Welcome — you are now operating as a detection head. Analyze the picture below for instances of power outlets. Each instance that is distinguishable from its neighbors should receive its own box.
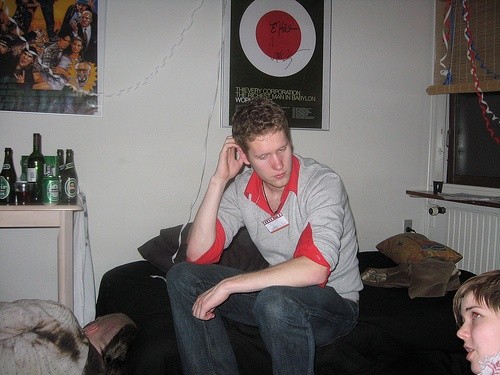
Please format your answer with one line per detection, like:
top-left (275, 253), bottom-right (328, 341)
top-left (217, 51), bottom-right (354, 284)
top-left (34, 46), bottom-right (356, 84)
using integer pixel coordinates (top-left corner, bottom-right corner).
top-left (405, 221), bottom-right (412, 235)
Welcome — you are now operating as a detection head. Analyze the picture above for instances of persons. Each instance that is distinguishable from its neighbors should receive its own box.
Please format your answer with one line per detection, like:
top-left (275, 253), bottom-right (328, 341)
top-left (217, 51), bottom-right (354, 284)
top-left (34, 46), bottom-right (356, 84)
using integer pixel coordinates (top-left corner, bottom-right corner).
top-left (166, 97), bottom-right (364, 375)
top-left (453, 270), bottom-right (500, 375)
top-left (0, 300), bottom-right (140, 375)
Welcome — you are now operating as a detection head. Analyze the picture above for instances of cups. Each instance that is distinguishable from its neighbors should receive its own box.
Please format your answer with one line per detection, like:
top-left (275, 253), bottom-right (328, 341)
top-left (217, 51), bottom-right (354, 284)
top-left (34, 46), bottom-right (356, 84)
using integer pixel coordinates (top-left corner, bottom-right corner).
top-left (433, 181), bottom-right (443, 194)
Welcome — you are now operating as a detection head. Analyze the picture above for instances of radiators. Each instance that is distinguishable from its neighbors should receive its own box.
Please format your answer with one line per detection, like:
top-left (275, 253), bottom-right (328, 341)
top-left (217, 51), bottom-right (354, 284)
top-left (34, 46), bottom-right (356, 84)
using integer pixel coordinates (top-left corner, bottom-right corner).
top-left (427, 199), bottom-right (500, 275)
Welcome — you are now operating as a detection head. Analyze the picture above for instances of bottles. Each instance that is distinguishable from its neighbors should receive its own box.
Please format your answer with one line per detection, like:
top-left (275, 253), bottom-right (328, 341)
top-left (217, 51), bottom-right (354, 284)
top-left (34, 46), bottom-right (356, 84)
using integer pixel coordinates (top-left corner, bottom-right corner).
top-left (63, 149), bottom-right (79, 205)
top-left (55, 148), bottom-right (64, 202)
top-left (25, 133), bottom-right (44, 203)
top-left (0, 147), bottom-right (16, 205)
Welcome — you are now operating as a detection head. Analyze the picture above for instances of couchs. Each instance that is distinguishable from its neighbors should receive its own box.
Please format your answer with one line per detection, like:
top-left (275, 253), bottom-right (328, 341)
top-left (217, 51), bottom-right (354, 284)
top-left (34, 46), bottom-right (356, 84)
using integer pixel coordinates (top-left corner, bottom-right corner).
top-left (90, 251), bottom-right (479, 375)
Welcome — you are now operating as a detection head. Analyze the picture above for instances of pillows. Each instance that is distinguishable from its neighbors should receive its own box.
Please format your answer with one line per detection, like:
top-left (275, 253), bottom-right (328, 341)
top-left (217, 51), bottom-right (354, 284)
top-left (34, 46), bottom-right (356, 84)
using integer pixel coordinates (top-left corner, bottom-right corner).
top-left (376, 233), bottom-right (463, 272)
top-left (135, 220), bottom-right (268, 278)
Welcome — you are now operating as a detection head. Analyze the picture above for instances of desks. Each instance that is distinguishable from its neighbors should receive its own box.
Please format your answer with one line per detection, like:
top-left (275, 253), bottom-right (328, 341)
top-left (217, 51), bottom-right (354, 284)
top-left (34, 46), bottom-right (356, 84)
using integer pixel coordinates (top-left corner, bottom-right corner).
top-left (0, 204), bottom-right (82, 314)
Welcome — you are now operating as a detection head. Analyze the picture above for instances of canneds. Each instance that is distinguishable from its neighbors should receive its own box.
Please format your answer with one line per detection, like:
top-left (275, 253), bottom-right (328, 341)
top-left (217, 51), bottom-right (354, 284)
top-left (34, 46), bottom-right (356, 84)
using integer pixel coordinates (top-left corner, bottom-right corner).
top-left (40, 175), bottom-right (60, 206)
